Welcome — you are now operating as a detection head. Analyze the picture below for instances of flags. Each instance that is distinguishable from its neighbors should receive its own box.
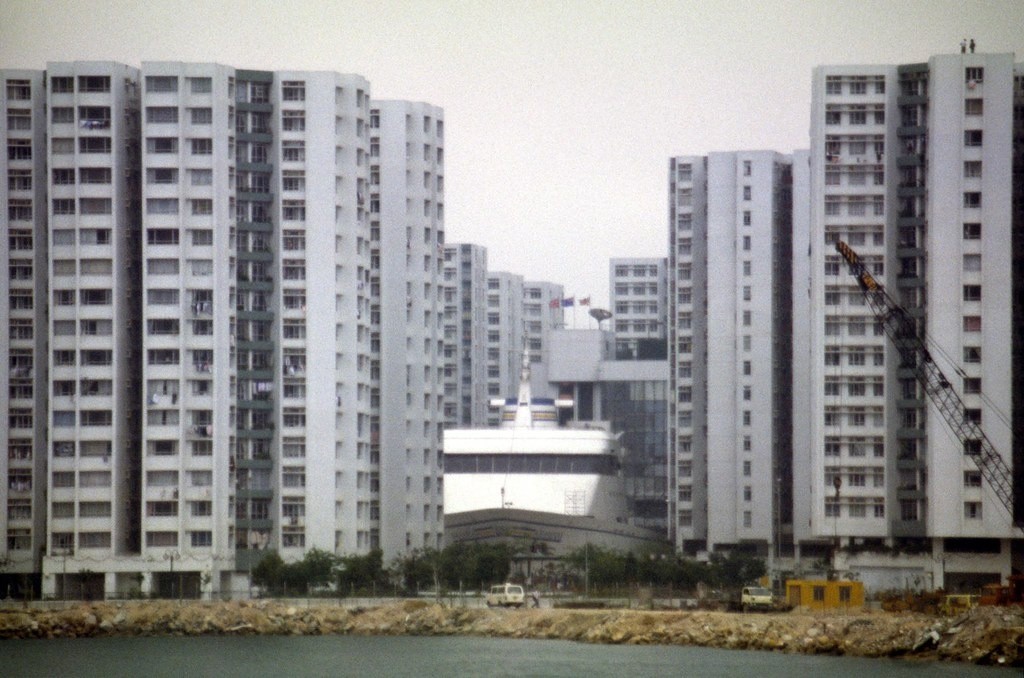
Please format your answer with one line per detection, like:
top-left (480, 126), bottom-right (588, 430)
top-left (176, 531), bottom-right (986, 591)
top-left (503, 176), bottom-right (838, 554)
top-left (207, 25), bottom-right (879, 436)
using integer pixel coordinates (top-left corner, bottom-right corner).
top-left (579, 297), bottom-right (590, 306)
top-left (561, 296), bottom-right (573, 306)
top-left (549, 299), bottom-right (559, 308)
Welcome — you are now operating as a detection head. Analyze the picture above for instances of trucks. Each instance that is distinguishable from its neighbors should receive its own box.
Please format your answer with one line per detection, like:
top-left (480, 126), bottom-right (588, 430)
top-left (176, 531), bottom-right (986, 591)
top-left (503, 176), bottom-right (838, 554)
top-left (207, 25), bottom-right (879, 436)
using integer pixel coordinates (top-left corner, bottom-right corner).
top-left (728, 585), bottom-right (773, 611)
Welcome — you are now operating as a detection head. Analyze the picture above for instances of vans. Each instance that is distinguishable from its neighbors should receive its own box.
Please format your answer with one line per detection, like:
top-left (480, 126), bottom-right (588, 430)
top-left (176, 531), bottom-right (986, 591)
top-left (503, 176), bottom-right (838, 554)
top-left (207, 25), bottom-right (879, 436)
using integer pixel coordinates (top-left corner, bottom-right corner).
top-left (485, 583), bottom-right (524, 608)
